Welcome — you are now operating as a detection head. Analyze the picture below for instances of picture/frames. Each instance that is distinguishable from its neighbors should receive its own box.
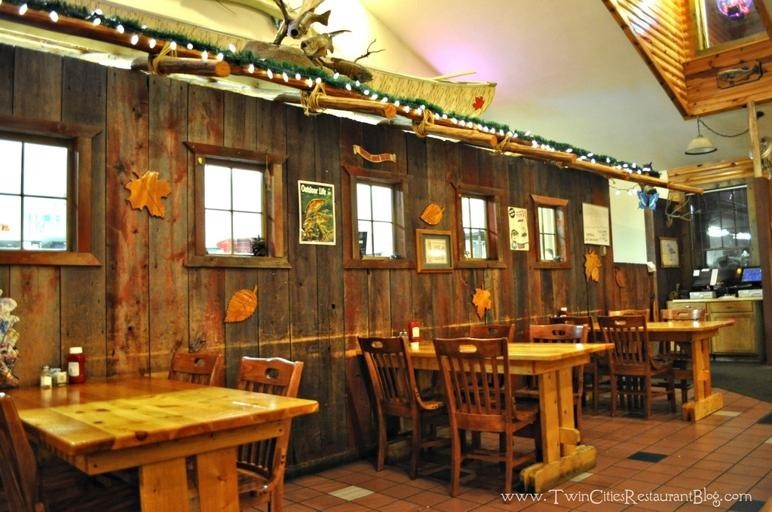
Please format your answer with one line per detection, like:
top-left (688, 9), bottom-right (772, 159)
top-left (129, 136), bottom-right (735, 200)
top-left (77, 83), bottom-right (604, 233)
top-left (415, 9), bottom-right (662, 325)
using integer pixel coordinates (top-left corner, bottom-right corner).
top-left (413, 226), bottom-right (456, 275)
top-left (657, 235), bottom-right (683, 270)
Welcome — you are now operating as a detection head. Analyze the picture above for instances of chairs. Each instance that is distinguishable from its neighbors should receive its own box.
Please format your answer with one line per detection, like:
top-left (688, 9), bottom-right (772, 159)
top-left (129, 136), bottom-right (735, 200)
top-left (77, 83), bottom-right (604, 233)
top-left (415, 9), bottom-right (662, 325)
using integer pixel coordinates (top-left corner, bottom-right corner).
top-left (462, 305), bottom-right (707, 445)
top-left (0, 391), bottom-right (138, 510)
top-left (355, 333), bottom-right (470, 480)
top-left (108, 350), bottom-right (225, 482)
top-left (231, 355), bottom-right (306, 512)
top-left (427, 337), bottom-right (544, 504)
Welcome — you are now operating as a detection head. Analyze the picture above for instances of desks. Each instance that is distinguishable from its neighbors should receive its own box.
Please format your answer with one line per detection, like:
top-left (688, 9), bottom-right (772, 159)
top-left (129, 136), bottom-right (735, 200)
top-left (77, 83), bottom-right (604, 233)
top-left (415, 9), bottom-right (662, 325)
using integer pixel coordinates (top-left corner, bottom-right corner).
top-left (577, 320), bottom-right (737, 423)
top-left (343, 340), bottom-right (617, 494)
top-left (3, 374), bottom-right (320, 512)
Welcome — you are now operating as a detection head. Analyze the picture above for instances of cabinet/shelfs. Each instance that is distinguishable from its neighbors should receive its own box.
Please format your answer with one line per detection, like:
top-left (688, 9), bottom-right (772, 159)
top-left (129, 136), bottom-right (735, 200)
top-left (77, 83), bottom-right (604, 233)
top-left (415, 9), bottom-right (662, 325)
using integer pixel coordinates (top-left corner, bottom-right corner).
top-left (665, 297), bottom-right (764, 356)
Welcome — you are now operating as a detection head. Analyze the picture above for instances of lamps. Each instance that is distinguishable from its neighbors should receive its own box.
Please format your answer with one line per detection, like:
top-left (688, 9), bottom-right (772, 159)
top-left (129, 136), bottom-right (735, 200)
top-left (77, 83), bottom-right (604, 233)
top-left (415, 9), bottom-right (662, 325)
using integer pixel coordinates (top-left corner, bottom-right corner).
top-left (681, 113), bottom-right (750, 157)
top-left (1, 1), bottom-right (659, 200)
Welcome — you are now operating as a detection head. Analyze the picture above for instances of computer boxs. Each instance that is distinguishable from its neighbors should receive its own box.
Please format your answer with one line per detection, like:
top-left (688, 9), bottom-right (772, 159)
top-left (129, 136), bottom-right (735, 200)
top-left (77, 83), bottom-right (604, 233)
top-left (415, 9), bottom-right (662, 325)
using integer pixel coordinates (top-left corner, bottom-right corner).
top-left (689, 292), bottom-right (717, 298)
top-left (738, 289), bottom-right (763, 298)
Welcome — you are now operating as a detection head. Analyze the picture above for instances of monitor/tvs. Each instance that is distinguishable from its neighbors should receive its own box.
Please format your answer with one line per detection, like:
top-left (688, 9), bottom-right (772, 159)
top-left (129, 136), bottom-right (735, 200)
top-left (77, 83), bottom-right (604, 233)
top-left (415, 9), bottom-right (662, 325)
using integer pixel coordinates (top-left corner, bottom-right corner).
top-left (689, 268), bottom-right (718, 290)
top-left (739, 266), bottom-right (762, 289)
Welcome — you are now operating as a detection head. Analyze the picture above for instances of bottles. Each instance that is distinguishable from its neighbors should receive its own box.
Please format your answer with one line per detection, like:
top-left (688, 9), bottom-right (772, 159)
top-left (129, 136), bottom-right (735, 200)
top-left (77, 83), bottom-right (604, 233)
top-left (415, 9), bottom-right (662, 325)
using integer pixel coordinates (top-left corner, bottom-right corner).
top-left (560, 307), bottom-right (569, 324)
top-left (399, 330), bottom-right (409, 351)
top-left (39, 366), bottom-right (52, 390)
top-left (40, 388), bottom-right (53, 408)
